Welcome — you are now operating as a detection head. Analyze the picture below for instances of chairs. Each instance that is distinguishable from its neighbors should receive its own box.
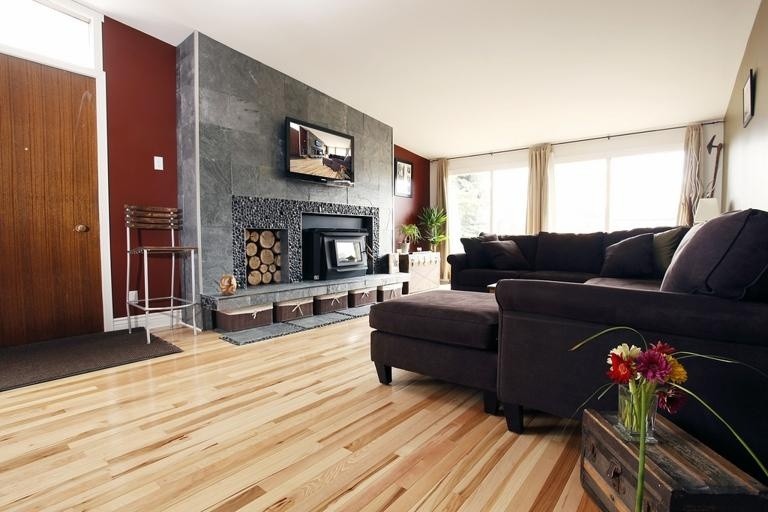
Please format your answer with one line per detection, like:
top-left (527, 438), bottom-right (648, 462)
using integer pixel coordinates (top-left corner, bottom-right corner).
top-left (123, 205), bottom-right (199, 344)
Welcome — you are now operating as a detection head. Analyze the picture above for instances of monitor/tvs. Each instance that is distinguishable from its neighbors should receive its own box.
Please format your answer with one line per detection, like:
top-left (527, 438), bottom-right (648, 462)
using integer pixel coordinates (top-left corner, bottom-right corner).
top-left (333, 239), bottom-right (364, 266)
top-left (284, 116), bottom-right (354, 191)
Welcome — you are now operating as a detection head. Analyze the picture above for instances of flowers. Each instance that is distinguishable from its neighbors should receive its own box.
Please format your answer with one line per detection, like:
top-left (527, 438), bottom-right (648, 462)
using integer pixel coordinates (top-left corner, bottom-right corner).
top-left (607, 341), bottom-right (686, 414)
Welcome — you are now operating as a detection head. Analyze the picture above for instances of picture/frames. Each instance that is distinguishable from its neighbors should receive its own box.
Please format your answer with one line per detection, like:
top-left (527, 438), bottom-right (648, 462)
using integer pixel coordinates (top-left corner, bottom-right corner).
top-left (394, 158), bottom-right (413, 198)
top-left (743, 68), bottom-right (753, 128)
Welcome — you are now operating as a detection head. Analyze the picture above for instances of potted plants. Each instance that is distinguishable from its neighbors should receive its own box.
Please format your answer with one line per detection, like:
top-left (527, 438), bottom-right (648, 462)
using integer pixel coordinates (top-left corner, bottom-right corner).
top-left (399, 224), bottom-right (425, 253)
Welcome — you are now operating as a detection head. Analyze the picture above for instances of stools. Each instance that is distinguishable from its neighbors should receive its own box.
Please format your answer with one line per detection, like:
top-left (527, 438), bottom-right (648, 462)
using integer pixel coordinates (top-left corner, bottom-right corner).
top-left (369, 290), bottom-right (498, 415)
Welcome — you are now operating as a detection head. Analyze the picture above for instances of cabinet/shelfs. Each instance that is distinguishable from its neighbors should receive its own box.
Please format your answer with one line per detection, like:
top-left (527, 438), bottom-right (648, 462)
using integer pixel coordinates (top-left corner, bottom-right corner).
top-left (396, 252), bottom-right (441, 294)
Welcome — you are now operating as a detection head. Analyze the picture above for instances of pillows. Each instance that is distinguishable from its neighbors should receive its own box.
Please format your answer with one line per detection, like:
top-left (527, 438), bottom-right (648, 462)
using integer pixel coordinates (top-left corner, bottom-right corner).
top-left (653, 226), bottom-right (682, 271)
top-left (460, 234), bottom-right (498, 265)
top-left (482, 240), bottom-right (531, 270)
top-left (601, 233), bottom-right (653, 277)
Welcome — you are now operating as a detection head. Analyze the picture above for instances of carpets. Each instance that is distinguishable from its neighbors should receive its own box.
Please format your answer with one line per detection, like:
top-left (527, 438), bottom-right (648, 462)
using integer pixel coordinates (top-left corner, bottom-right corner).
top-left (0, 327), bottom-right (183, 393)
top-left (219, 303), bottom-right (376, 346)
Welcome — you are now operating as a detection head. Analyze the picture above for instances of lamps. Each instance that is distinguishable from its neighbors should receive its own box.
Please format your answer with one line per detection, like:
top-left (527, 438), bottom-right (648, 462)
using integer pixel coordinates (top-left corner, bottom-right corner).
top-left (695, 198), bottom-right (720, 223)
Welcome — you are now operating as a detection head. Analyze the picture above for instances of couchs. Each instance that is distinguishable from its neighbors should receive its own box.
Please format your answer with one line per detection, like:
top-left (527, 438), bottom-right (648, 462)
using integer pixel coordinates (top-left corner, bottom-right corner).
top-left (447, 209), bottom-right (768, 432)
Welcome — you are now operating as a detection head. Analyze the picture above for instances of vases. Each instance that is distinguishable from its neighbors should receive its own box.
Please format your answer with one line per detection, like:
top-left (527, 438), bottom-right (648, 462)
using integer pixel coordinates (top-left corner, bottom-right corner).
top-left (614, 382), bottom-right (659, 442)
